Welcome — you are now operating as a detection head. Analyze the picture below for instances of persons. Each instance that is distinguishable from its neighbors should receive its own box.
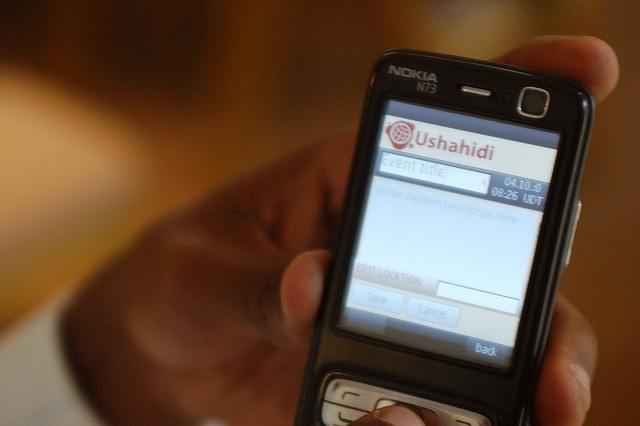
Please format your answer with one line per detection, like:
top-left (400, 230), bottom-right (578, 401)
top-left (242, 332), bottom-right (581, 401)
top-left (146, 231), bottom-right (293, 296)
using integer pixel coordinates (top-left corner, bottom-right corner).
top-left (1, 34), bottom-right (620, 426)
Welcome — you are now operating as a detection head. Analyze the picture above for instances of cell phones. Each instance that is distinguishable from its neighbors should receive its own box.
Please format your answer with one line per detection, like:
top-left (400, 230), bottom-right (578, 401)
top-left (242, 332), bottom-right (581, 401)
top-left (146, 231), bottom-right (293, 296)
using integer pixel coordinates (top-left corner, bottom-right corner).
top-left (294, 48), bottom-right (596, 426)
